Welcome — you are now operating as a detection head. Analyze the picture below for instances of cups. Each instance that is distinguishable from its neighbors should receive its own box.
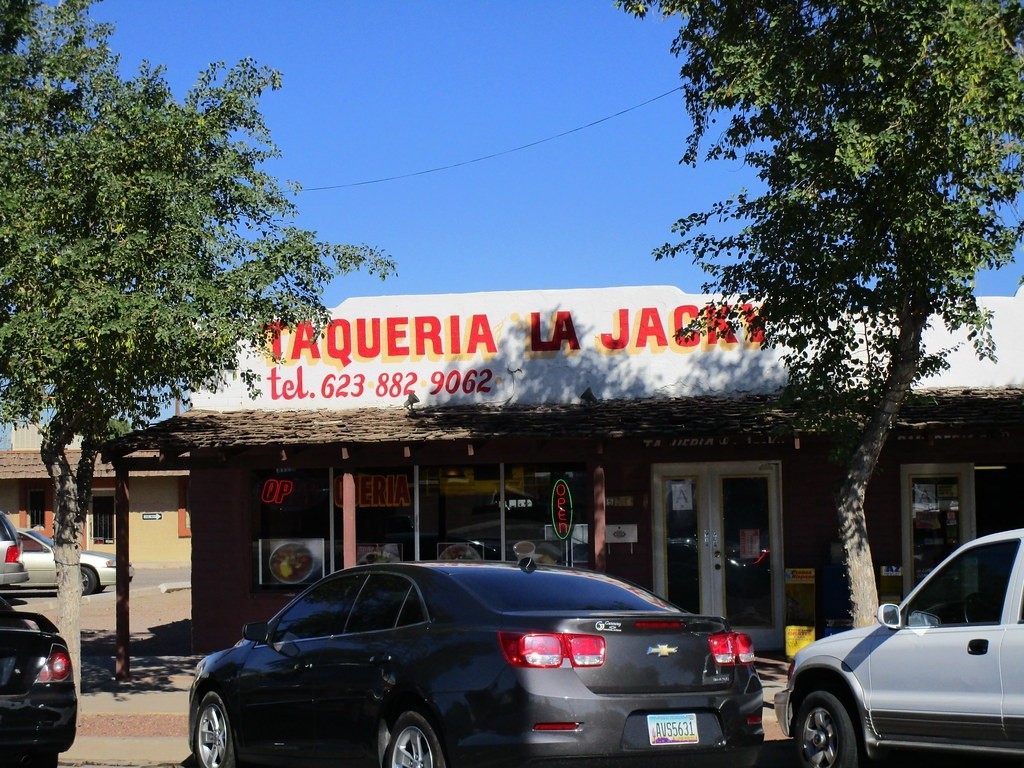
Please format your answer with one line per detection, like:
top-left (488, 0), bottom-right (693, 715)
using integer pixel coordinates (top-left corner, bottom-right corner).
top-left (513, 541), bottom-right (536, 561)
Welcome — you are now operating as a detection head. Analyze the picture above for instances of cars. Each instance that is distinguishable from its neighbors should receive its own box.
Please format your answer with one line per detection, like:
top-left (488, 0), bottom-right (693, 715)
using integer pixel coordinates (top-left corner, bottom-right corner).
top-left (0, 512), bottom-right (29, 585)
top-left (0, 598), bottom-right (78, 768)
top-left (188, 563), bottom-right (765, 768)
top-left (16, 530), bottom-right (134, 595)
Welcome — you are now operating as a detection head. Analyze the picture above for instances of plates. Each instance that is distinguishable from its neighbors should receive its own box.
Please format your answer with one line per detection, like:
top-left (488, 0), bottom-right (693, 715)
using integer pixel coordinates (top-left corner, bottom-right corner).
top-left (357, 551), bottom-right (400, 566)
top-left (268, 542), bottom-right (315, 584)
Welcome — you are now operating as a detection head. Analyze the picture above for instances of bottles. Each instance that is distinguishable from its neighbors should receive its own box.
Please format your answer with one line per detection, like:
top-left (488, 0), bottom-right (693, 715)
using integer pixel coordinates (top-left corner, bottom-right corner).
top-left (439, 544), bottom-right (481, 560)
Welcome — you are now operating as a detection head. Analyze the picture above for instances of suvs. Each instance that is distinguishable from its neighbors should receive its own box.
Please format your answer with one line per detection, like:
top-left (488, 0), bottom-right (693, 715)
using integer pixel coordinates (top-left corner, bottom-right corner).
top-left (776, 529), bottom-right (1022, 768)
